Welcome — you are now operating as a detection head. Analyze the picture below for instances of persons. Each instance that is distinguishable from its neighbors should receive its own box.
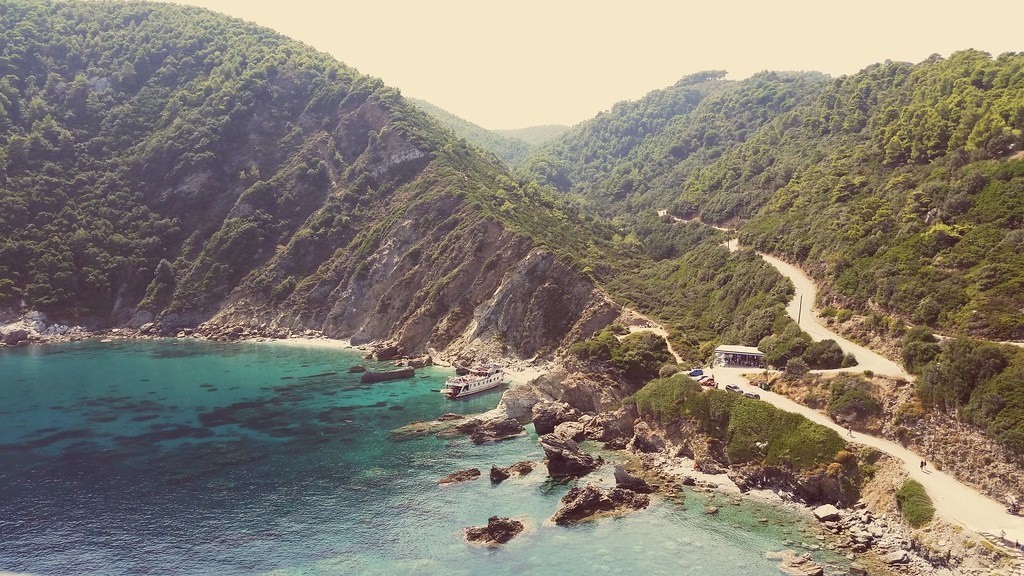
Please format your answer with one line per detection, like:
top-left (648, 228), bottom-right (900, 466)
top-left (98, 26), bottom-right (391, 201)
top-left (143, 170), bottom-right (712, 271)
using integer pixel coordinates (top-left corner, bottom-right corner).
top-left (920, 460), bottom-right (924, 471)
top-left (846, 426), bottom-right (852, 437)
top-left (923, 460), bottom-right (927, 471)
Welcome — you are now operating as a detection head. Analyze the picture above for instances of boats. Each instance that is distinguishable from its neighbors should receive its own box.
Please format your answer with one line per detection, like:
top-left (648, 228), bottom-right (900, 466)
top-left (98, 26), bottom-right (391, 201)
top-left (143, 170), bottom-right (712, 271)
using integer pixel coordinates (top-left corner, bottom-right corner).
top-left (442, 363), bottom-right (503, 399)
